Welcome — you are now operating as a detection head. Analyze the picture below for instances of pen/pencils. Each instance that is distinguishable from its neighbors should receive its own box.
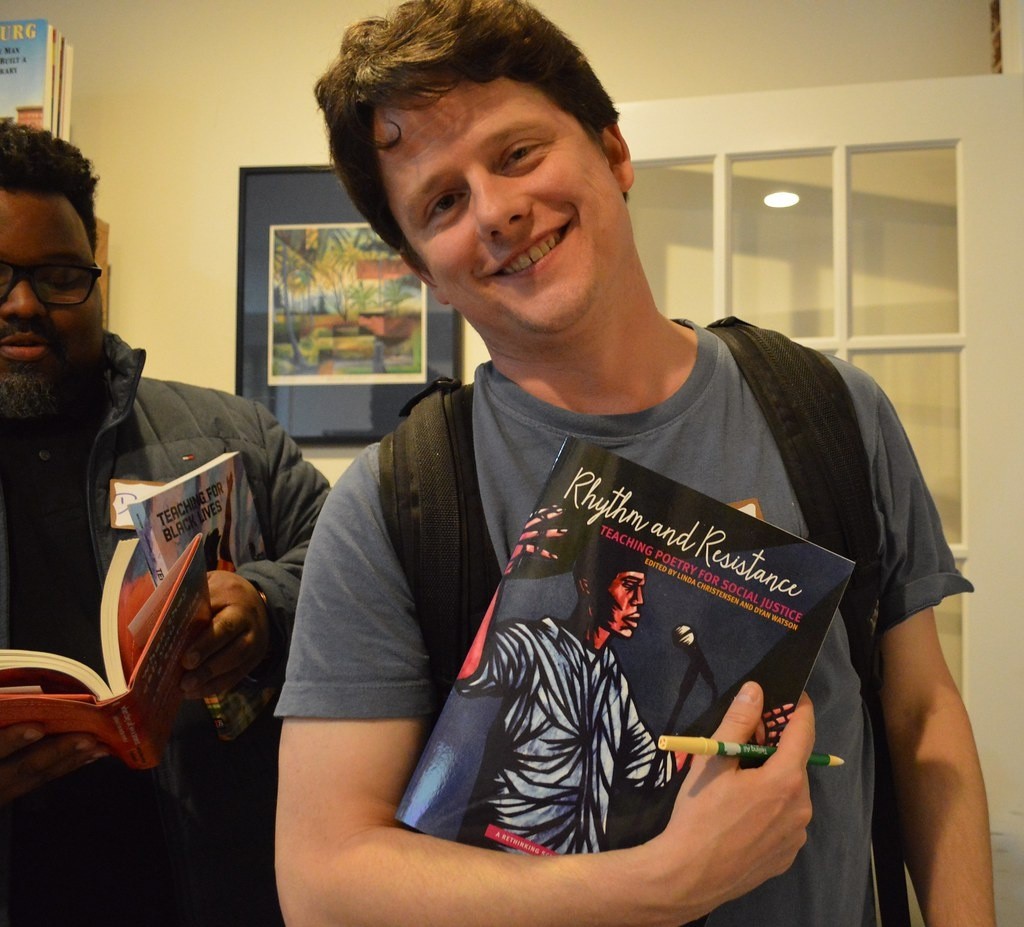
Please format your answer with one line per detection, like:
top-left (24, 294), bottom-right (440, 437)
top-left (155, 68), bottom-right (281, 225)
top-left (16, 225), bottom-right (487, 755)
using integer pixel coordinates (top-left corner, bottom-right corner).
top-left (658, 735), bottom-right (845, 768)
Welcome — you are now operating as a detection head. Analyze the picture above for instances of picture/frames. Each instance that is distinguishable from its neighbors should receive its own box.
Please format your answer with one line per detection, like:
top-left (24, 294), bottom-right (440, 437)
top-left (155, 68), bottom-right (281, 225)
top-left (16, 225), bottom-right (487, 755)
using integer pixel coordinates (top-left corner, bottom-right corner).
top-left (234, 164), bottom-right (463, 447)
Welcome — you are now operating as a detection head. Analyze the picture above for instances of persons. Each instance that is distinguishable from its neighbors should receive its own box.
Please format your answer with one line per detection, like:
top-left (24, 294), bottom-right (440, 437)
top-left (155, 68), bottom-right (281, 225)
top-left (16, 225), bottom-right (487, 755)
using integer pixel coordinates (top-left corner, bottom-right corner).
top-left (0, 119), bottom-right (334, 927)
top-left (273, 1), bottom-right (997, 927)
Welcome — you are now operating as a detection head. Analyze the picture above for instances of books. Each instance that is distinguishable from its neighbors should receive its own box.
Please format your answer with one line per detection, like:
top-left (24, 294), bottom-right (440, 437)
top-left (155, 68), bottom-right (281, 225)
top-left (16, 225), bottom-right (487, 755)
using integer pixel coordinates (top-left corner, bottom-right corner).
top-left (0, 441), bottom-right (280, 770)
top-left (397, 437), bottom-right (854, 927)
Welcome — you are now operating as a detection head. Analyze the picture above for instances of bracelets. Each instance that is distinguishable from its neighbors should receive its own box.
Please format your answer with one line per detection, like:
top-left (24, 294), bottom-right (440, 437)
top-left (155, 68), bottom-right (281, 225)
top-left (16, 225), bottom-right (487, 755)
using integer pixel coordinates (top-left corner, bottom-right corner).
top-left (258, 591), bottom-right (269, 607)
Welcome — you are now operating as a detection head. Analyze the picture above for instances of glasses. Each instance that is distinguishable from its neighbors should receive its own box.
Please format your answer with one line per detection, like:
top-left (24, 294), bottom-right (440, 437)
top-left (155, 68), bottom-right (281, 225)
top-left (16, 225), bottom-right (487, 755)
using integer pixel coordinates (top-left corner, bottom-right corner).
top-left (0, 260), bottom-right (102, 305)
